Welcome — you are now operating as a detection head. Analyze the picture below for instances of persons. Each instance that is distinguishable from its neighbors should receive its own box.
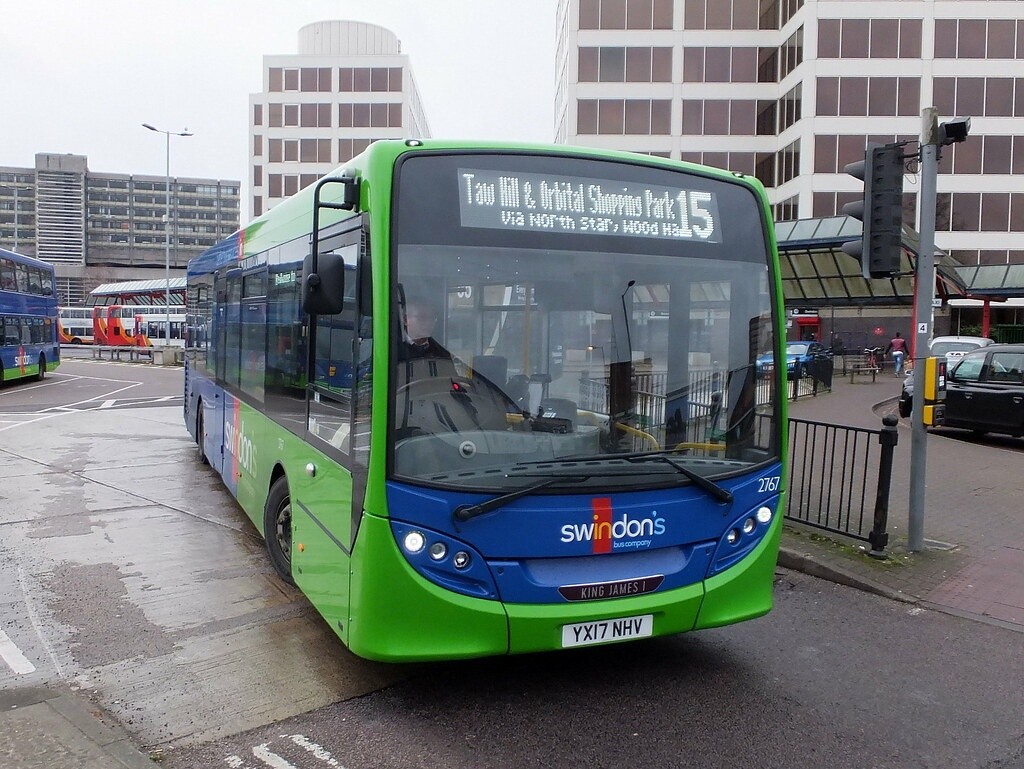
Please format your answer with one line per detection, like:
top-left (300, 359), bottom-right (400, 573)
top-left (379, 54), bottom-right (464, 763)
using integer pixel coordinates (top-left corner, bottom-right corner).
top-left (379, 292), bottom-right (483, 448)
top-left (883, 331), bottom-right (912, 379)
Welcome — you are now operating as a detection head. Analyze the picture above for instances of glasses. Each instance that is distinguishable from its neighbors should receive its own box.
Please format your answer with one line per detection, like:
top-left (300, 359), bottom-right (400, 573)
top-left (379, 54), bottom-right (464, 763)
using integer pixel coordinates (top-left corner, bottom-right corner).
top-left (407, 314), bottom-right (438, 323)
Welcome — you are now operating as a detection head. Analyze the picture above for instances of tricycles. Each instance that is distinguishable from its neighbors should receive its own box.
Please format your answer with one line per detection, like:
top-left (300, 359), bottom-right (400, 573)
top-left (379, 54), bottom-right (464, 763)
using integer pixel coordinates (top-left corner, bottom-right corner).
top-left (847, 347), bottom-right (883, 383)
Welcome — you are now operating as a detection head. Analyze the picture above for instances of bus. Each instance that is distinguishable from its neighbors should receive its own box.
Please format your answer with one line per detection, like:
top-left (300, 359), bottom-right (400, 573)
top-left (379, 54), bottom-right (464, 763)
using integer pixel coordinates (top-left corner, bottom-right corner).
top-left (0, 247), bottom-right (61, 384)
top-left (133, 312), bottom-right (186, 352)
top-left (55, 306), bottom-right (94, 345)
top-left (92, 305), bottom-right (109, 346)
top-left (106, 303), bottom-right (187, 345)
top-left (183, 133), bottom-right (796, 668)
top-left (214, 257), bottom-right (373, 402)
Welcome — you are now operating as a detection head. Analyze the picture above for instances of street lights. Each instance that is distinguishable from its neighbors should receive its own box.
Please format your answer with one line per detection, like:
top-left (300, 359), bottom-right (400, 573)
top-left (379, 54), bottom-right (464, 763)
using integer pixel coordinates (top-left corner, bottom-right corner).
top-left (587, 343), bottom-right (607, 374)
top-left (620, 280), bottom-right (637, 366)
top-left (142, 122), bottom-right (196, 347)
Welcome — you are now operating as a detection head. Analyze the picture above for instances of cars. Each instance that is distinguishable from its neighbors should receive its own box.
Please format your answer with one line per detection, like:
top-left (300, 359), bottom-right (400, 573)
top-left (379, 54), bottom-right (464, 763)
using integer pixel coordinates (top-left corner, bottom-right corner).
top-left (755, 339), bottom-right (830, 382)
top-left (903, 336), bottom-right (994, 394)
top-left (898, 344), bottom-right (1024, 447)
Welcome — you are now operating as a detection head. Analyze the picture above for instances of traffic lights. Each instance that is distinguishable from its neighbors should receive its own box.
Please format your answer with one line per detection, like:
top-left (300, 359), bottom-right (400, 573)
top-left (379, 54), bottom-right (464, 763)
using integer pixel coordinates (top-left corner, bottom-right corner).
top-left (844, 141), bottom-right (904, 282)
